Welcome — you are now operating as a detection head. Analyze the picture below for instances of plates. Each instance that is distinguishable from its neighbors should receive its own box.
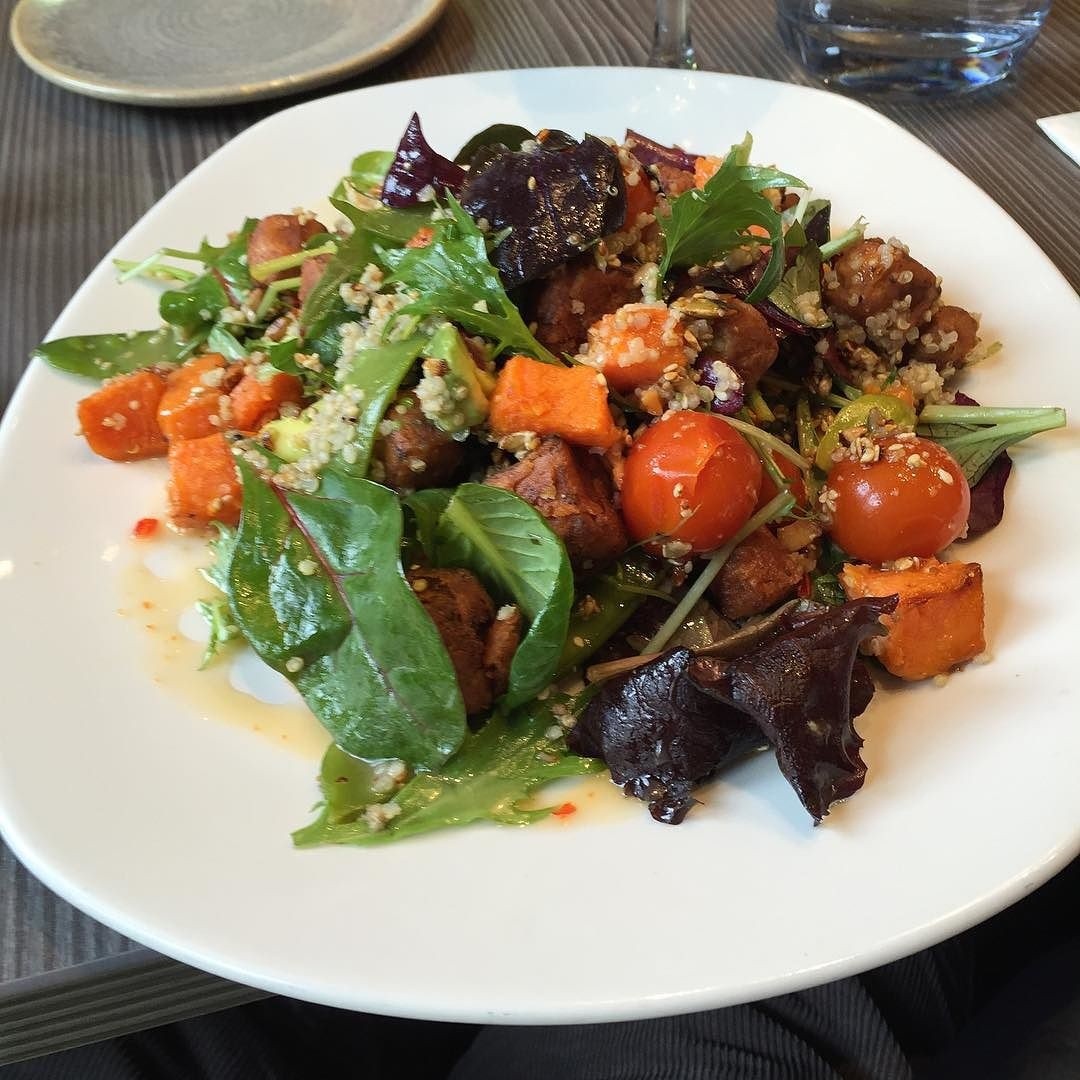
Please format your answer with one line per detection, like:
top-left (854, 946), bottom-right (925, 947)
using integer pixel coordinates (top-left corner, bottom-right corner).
top-left (7, 0), bottom-right (449, 108)
top-left (0, 65), bottom-right (1080, 1026)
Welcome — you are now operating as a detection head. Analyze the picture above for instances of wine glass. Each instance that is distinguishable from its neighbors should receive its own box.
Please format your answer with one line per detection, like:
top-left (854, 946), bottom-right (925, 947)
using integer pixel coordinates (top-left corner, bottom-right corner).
top-left (644, 0), bottom-right (703, 71)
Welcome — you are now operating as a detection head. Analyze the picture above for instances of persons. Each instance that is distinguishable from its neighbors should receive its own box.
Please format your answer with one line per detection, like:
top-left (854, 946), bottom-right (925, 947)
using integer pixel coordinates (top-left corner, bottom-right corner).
top-left (0, 939), bottom-right (972, 1080)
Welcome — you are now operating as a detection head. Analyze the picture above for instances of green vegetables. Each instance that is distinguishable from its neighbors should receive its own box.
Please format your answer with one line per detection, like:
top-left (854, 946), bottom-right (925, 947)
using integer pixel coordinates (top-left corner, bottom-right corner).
top-left (21, 123), bottom-right (1068, 870)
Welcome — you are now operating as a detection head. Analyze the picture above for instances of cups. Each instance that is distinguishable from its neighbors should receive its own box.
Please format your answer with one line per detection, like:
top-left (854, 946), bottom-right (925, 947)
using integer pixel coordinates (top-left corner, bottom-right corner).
top-left (774, 0), bottom-right (1050, 97)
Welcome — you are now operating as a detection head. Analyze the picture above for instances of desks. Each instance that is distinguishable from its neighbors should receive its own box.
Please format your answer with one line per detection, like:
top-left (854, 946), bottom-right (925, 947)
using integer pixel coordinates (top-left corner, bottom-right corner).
top-left (1, 0), bottom-right (1080, 1070)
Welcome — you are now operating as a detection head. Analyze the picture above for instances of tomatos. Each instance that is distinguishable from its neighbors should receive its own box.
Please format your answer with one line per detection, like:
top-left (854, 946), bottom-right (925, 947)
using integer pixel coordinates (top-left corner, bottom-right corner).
top-left (816, 433), bottom-right (971, 566)
top-left (759, 437), bottom-right (807, 525)
top-left (615, 409), bottom-right (759, 557)
